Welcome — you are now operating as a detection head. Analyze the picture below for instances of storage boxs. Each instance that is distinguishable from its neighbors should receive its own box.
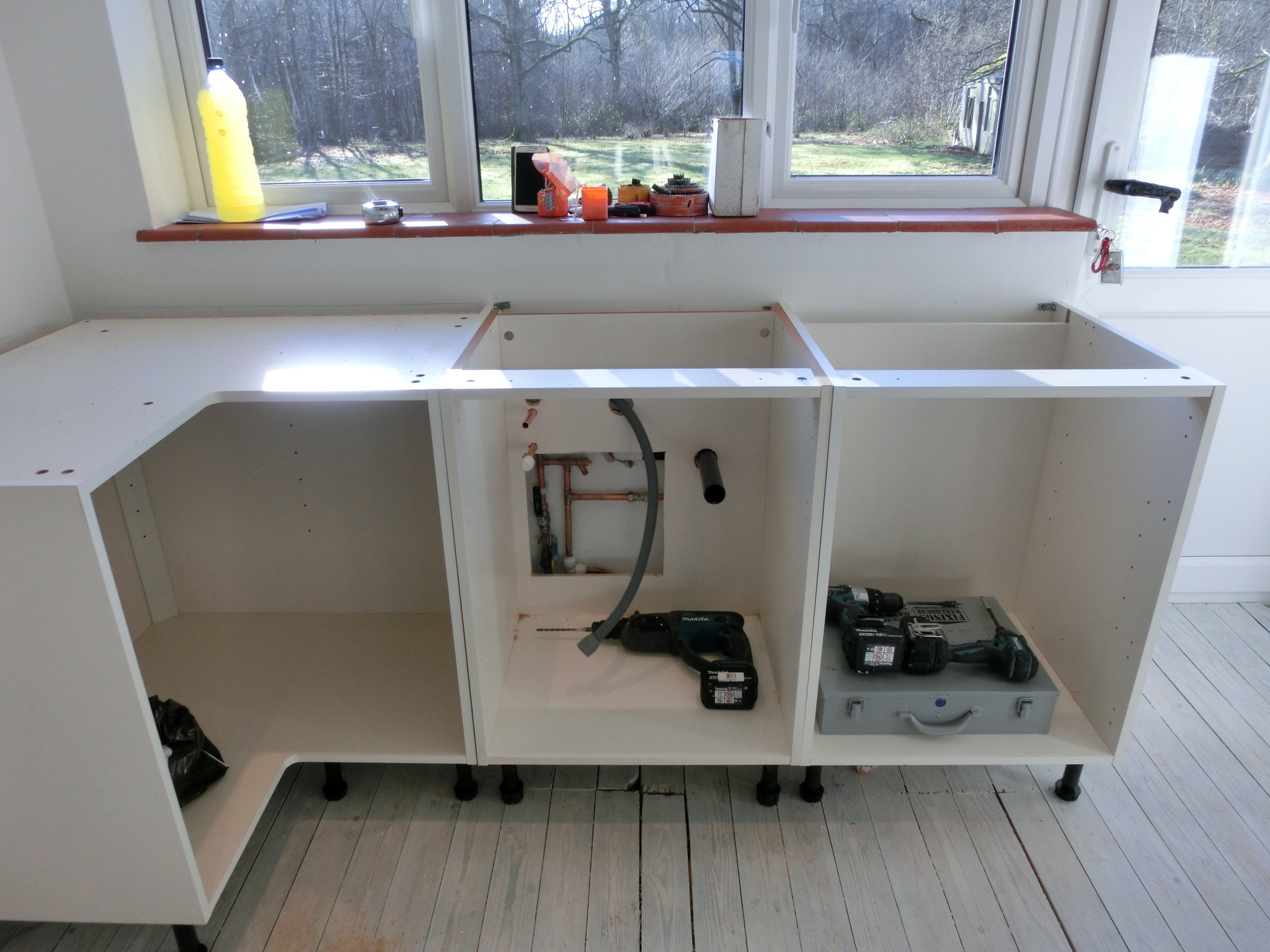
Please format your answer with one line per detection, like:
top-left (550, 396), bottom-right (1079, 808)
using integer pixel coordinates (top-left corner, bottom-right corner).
top-left (709, 115), bottom-right (765, 216)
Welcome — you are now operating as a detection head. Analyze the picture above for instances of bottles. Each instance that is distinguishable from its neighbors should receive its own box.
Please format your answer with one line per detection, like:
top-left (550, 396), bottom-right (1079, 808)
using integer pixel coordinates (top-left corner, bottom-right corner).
top-left (196, 57), bottom-right (266, 222)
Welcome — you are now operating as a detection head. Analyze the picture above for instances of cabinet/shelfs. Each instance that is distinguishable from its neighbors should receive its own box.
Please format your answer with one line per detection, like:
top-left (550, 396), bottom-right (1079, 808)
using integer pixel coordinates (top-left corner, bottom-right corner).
top-left (434, 305), bottom-right (834, 808)
top-left (0, 304), bottom-right (491, 952)
top-left (794, 301), bottom-right (1226, 804)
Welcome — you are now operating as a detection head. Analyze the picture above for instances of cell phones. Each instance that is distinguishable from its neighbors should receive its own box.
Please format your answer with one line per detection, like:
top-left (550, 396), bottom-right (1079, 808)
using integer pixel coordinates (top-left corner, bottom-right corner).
top-left (512, 146), bottom-right (549, 213)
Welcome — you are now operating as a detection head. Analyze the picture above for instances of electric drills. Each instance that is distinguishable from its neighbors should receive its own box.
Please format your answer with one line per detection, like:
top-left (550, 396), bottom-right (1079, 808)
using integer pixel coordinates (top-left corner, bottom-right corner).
top-left (535, 609), bottom-right (759, 710)
top-left (842, 597), bottom-right (1039, 682)
top-left (825, 584), bottom-right (962, 623)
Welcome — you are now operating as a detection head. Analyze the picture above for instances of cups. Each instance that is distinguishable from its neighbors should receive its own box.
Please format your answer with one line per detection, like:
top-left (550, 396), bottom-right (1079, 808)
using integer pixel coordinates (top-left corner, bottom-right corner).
top-left (581, 186), bottom-right (609, 221)
